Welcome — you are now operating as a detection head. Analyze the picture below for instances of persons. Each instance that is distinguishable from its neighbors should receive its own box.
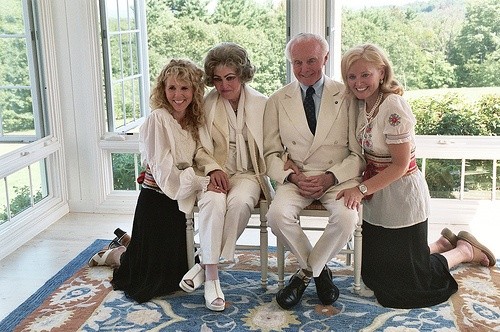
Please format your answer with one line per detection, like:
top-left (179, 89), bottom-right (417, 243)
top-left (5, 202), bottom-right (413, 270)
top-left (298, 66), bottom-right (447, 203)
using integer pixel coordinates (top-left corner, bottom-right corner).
top-left (179, 41), bottom-right (299, 311)
top-left (263, 32), bottom-right (367, 310)
top-left (88, 59), bottom-right (227, 303)
top-left (336, 44), bottom-right (496, 309)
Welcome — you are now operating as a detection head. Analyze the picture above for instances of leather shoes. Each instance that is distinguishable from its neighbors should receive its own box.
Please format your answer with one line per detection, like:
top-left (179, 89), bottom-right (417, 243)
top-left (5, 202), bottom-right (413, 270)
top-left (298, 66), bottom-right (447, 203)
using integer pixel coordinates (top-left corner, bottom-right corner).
top-left (276, 269), bottom-right (311, 310)
top-left (314, 264), bottom-right (339, 305)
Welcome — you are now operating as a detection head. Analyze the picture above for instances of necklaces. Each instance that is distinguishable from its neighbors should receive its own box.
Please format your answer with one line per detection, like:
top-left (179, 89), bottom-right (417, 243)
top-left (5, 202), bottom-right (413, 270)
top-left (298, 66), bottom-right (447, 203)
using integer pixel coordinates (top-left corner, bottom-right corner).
top-left (233, 107), bottom-right (238, 111)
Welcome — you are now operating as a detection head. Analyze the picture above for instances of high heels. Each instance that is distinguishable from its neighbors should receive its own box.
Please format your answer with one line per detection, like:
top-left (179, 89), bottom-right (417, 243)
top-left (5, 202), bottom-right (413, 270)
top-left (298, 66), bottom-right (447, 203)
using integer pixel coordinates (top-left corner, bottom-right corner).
top-left (109, 228), bottom-right (130, 249)
top-left (88, 241), bottom-right (122, 267)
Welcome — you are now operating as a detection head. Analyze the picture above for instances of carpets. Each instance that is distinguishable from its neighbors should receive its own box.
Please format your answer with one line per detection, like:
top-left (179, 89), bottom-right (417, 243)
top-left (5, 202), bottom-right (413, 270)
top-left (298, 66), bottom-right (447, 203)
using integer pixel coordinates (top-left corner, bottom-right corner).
top-left (0, 239), bottom-right (500, 332)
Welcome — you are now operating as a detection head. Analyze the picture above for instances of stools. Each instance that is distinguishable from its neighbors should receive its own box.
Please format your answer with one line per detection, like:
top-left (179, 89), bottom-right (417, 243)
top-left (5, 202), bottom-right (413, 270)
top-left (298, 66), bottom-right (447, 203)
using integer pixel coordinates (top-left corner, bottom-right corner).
top-left (185, 194), bottom-right (269, 287)
top-left (277, 201), bottom-right (364, 294)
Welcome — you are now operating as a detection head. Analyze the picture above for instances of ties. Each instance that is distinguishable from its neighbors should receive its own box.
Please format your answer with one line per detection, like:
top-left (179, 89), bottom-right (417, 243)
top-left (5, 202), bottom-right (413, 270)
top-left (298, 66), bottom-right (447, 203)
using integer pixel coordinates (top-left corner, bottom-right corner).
top-left (303, 87), bottom-right (317, 136)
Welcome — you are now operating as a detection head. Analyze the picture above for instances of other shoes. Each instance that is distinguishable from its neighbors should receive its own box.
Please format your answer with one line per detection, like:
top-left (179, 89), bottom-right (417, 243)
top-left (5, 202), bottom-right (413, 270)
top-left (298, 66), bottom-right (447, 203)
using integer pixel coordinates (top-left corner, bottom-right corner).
top-left (204, 278), bottom-right (225, 311)
top-left (179, 263), bottom-right (204, 292)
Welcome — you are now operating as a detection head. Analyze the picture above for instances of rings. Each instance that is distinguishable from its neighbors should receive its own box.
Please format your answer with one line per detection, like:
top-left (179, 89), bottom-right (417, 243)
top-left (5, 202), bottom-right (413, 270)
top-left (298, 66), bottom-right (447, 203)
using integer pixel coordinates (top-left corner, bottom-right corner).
top-left (215, 186), bottom-right (219, 189)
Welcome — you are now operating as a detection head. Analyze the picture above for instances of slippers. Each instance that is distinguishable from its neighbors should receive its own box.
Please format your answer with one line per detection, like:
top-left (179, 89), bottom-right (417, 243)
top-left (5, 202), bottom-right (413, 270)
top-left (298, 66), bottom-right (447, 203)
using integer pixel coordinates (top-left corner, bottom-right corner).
top-left (441, 228), bottom-right (458, 248)
top-left (458, 231), bottom-right (496, 266)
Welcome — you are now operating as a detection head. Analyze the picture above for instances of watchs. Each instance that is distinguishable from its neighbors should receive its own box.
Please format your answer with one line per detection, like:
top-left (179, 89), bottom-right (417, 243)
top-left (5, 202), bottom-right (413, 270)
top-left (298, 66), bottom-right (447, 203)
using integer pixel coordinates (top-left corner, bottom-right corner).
top-left (357, 183), bottom-right (368, 196)
